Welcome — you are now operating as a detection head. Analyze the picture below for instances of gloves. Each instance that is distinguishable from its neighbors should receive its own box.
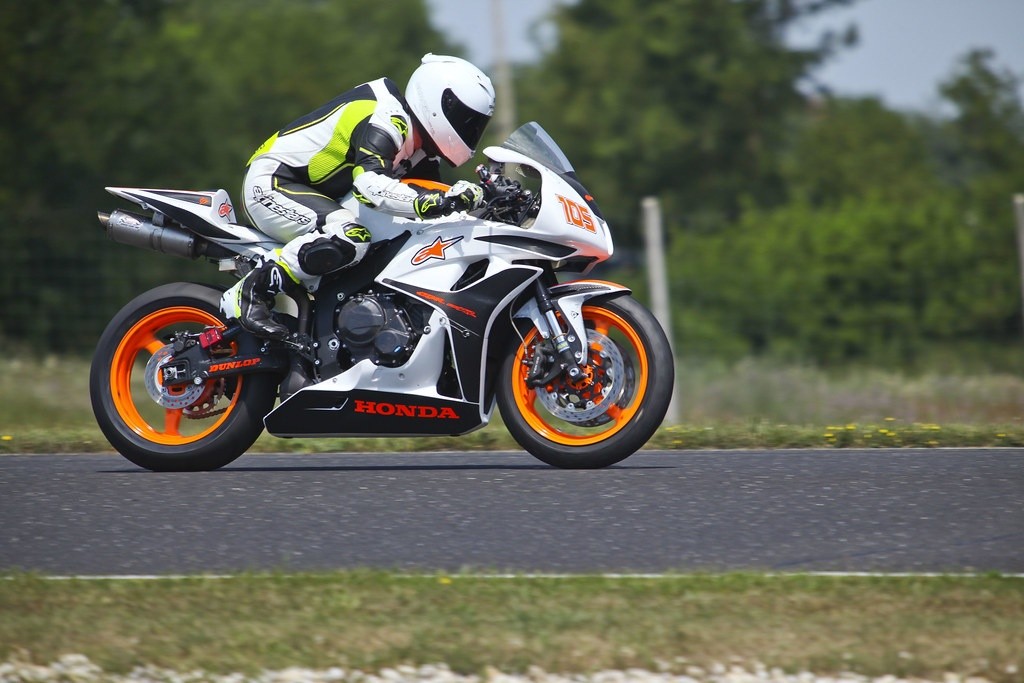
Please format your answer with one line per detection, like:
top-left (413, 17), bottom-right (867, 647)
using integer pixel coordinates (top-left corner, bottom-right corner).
top-left (413, 180), bottom-right (486, 221)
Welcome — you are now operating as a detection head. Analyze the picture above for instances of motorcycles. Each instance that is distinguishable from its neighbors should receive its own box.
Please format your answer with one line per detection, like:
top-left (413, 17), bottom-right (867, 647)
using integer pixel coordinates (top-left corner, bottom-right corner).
top-left (88, 120), bottom-right (675, 472)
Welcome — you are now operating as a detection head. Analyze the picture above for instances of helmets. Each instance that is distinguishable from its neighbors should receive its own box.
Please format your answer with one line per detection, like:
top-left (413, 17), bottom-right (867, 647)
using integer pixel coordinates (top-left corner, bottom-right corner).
top-left (403, 52), bottom-right (496, 168)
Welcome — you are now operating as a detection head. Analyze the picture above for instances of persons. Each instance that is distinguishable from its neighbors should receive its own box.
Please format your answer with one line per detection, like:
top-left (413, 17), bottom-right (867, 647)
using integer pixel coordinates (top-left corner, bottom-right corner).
top-left (219, 52), bottom-right (496, 340)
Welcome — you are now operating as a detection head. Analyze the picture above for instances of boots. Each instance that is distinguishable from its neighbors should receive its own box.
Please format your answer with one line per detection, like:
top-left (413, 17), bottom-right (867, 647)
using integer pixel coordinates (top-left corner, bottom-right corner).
top-left (219, 256), bottom-right (301, 342)
